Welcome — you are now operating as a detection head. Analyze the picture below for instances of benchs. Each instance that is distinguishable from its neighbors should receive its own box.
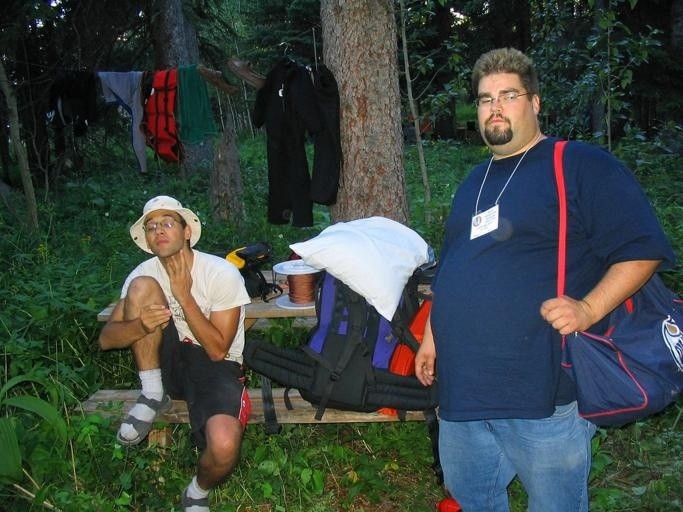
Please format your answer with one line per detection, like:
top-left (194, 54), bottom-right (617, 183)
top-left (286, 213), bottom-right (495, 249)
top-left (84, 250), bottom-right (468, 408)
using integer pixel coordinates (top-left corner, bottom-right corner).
top-left (77, 388), bottom-right (444, 496)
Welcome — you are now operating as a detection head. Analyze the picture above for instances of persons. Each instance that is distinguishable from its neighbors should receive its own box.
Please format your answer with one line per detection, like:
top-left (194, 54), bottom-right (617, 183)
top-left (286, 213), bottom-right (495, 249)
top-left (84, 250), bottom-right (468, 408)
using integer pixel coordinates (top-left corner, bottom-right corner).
top-left (98, 194), bottom-right (250, 512)
top-left (412, 47), bottom-right (671, 512)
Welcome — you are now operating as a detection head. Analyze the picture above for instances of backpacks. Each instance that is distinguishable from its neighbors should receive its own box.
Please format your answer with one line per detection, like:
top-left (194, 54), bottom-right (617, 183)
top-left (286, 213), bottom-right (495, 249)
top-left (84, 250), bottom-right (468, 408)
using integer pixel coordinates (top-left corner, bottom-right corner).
top-left (297, 266), bottom-right (422, 413)
top-left (222, 243), bottom-right (272, 303)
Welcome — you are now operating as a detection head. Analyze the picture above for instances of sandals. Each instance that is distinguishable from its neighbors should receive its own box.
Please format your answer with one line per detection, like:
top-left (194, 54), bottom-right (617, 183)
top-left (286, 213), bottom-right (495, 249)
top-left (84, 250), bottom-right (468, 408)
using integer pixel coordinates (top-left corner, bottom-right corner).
top-left (116, 387), bottom-right (173, 446)
top-left (181, 488), bottom-right (212, 512)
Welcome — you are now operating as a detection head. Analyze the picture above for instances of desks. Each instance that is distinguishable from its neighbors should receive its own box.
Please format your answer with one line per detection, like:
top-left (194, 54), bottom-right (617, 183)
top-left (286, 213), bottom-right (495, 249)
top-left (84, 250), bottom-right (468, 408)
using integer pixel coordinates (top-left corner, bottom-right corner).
top-left (96, 270), bottom-right (437, 334)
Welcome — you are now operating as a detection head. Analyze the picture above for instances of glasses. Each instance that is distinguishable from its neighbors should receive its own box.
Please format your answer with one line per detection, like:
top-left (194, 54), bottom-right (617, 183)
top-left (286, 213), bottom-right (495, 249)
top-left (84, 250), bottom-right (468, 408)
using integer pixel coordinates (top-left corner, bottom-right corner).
top-left (475, 91), bottom-right (535, 105)
top-left (142, 218), bottom-right (185, 229)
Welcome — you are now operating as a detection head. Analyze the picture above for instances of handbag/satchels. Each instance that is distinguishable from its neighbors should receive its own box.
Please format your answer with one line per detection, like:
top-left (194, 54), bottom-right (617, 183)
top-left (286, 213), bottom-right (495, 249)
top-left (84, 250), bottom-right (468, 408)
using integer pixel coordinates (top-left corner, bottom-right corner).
top-left (564, 276), bottom-right (682, 429)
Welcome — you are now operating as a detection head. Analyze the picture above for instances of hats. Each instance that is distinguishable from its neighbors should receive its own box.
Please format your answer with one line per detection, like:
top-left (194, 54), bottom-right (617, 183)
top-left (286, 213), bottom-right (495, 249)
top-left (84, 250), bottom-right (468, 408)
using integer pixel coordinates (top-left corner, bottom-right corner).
top-left (130, 195), bottom-right (202, 255)
